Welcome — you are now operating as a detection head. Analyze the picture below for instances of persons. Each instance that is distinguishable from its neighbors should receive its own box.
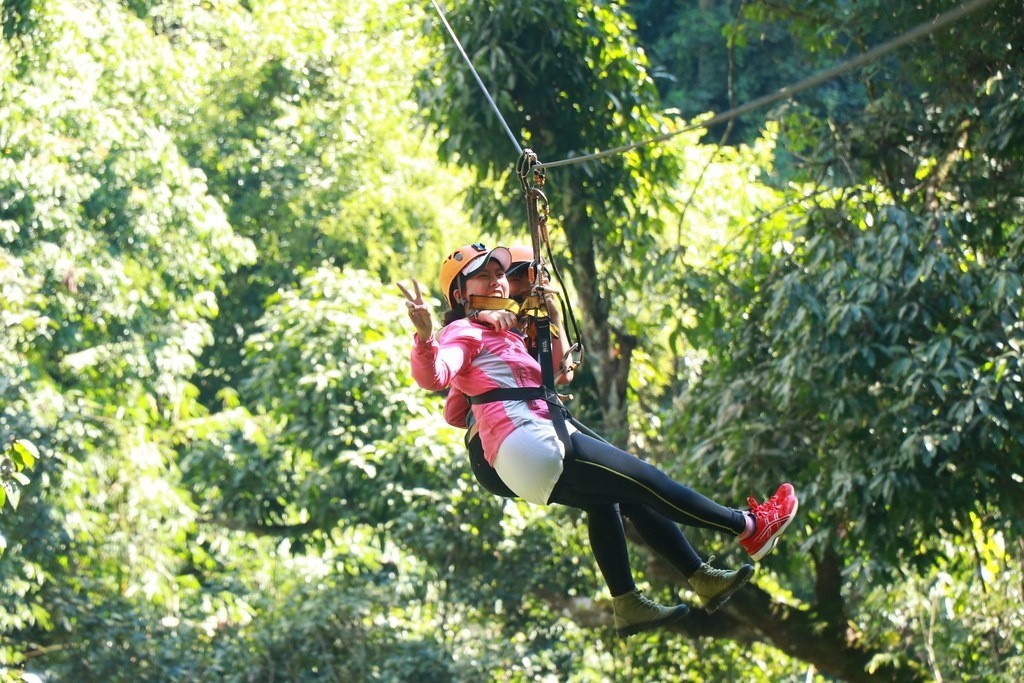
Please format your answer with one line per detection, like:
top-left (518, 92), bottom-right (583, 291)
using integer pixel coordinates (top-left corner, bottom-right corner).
top-left (444, 247), bottom-right (755, 639)
top-left (396, 243), bottom-right (799, 562)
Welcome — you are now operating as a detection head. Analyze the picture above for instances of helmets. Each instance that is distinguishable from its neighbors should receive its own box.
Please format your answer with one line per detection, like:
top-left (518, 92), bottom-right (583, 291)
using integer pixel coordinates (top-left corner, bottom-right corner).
top-left (439, 243), bottom-right (488, 310)
top-left (508, 246), bottom-right (543, 264)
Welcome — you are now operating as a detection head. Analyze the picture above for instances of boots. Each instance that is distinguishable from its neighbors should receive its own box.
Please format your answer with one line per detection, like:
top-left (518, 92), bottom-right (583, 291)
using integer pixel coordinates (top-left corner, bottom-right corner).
top-left (688, 555), bottom-right (755, 615)
top-left (612, 587), bottom-right (689, 639)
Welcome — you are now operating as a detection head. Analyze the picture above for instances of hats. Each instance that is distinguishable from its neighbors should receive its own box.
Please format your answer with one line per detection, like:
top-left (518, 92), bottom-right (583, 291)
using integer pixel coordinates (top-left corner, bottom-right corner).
top-left (451, 245), bottom-right (512, 303)
top-left (505, 261), bottom-right (551, 282)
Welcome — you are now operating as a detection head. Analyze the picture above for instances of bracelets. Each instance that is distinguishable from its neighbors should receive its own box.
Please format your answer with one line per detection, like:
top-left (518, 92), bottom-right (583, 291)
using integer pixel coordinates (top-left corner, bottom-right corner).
top-left (475, 310), bottom-right (483, 320)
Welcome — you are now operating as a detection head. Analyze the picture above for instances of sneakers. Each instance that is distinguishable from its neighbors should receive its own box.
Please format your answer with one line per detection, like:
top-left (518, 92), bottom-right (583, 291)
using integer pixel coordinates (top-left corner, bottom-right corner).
top-left (740, 495), bottom-right (799, 561)
top-left (747, 483), bottom-right (795, 556)
